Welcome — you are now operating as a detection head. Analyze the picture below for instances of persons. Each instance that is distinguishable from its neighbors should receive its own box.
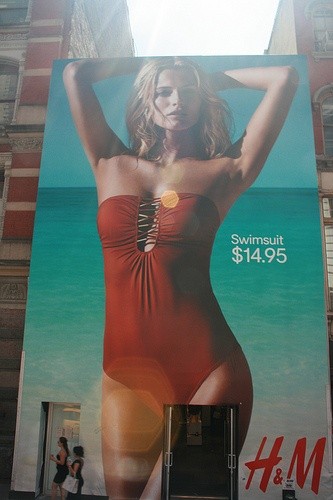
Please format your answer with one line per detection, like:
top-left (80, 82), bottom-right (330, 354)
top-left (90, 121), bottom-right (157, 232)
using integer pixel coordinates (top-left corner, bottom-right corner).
top-left (48, 436), bottom-right (71, 500)
top-left (67, 446), bottom-right (85, 500)
top-left (60, 57), bottom-right (299, 500)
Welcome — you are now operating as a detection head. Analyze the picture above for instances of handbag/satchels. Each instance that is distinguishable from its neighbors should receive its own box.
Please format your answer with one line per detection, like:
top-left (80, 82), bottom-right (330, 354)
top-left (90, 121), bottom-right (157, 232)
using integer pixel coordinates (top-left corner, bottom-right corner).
top-left (62, 469), bottom-right (79, 494)
